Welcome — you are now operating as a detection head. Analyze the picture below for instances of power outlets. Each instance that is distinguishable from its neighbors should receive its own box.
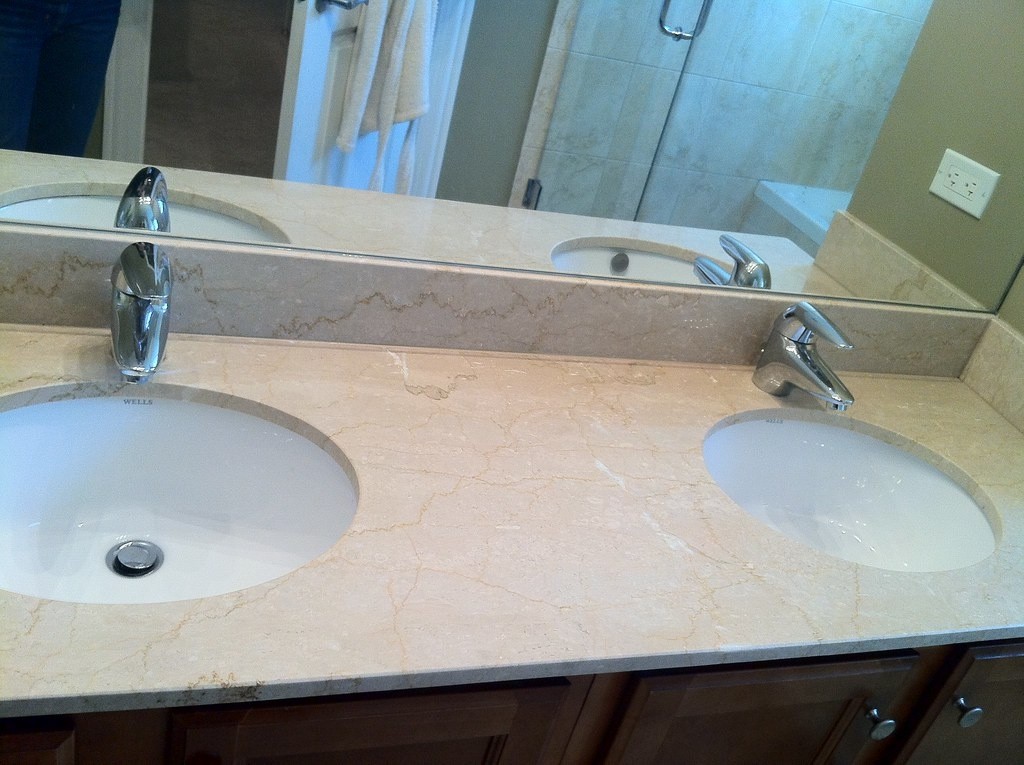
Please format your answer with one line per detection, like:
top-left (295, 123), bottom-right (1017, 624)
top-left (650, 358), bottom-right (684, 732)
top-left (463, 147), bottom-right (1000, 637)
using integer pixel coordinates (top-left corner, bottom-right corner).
top-left (927, 147), bottom-right (1001, 221)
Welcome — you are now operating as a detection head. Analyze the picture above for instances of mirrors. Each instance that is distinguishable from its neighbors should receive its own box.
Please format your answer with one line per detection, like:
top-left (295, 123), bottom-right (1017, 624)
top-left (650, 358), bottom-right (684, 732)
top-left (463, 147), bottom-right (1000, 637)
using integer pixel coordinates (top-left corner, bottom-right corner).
top-left (0, 0), bottom-right (1024, 312)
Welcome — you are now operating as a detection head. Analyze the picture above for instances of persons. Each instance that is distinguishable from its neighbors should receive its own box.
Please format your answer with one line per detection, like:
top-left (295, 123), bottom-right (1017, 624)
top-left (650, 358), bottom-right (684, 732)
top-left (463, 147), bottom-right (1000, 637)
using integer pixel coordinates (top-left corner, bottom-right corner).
top-left (0, 0), bottom-right (122, 157)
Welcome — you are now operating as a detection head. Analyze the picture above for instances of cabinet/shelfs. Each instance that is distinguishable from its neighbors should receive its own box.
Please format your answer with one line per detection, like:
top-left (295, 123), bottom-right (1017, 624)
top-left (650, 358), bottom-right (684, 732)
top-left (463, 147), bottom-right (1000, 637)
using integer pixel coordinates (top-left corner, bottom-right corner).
top-left (0, 636), bottom-right (1023, 765)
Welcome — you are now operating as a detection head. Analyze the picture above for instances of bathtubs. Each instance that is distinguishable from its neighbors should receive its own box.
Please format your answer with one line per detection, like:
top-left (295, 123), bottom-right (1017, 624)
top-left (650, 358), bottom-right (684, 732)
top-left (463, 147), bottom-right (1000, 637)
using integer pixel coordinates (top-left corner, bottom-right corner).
top-left (739, 177), bottom-right (857, 262)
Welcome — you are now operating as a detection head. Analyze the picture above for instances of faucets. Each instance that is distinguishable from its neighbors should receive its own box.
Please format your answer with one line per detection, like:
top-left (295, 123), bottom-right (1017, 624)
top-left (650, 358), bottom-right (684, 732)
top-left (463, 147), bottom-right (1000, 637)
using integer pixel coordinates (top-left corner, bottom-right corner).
top-left (692, 233), bottom-right (772, 291)
top-left (109, 241), bottom-right (172, 385)
top-left (752, 301), bottom-right (855, 413)
top-left (116, 166), bottom-right (171, 233)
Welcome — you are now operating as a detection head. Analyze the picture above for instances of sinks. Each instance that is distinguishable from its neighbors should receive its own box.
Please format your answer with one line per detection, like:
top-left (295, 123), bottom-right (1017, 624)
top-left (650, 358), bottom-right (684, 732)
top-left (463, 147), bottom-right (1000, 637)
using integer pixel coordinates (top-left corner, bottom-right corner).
top-left (702, 406), bottom-right (1002, 574)
top-left (548, 235), bottom-right (747, 287)
top-left (0, 381), bottom-right (361, 602)
top-left (1, 181), bottom-right (289, 248)
